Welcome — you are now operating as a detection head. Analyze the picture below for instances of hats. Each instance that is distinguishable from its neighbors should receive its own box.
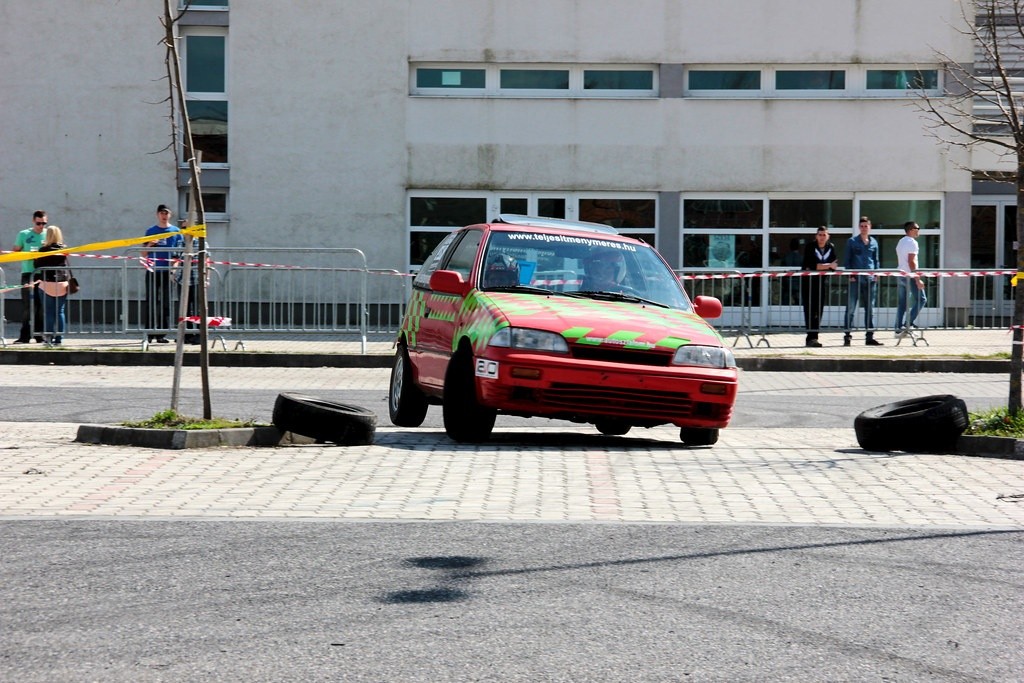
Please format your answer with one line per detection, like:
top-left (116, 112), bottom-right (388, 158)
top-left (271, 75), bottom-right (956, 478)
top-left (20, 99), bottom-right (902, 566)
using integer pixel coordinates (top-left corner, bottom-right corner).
top-left (158, 204), bottom-right (171, 213)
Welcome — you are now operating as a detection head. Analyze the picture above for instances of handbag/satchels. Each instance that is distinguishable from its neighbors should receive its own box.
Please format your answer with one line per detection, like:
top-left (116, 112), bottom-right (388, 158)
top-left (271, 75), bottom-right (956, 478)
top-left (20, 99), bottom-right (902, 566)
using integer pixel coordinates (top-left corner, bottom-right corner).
top-left (69, 277), bottom-right (80, 294)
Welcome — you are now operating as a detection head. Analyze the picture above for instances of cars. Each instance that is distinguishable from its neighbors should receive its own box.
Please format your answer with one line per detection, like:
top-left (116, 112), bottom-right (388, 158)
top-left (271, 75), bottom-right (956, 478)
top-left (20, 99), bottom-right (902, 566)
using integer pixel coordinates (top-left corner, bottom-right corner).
top-left (386, 214), bottom-right (740, 448)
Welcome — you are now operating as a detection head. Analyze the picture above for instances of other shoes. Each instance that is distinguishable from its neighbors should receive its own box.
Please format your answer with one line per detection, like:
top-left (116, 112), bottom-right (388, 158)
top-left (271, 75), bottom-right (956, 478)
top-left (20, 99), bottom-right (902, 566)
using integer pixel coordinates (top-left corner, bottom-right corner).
top-left (844, 340), bottom-right (851, 346)
top-left (806, 339), bottom-right (822, 347)
top-left (174, 332), bottom-right (202, 346)
top-left (142, 339), bottom-right (151, 343)
top-left (14, 338), bottom-right (30, 344)
top-left (36, 339), bottom-right (47, 343)
top-left (866, 339), bottom-right (882, 345)
top-left (157, 338), bottom-right (169, 343)
top-left (903, 321), bottom-right (921, 331)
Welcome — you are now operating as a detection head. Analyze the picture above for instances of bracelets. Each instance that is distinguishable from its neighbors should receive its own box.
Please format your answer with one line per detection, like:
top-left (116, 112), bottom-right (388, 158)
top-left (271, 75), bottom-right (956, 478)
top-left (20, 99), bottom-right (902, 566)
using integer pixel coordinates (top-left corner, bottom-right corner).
top-left (170, 272), bottom-right (173, 274)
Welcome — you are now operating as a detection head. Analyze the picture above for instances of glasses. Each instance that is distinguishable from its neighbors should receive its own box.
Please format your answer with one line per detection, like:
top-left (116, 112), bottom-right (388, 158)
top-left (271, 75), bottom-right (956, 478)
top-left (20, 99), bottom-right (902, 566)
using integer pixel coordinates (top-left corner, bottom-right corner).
top-left (913, 228), bottom-right (920, 230)
top-left (36, 221), bottom-right (47, 226)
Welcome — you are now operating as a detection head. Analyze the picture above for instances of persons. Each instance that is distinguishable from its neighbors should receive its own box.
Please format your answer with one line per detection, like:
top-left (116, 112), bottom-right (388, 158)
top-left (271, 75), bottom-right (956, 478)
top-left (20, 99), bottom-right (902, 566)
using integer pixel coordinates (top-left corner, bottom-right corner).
top-left (579, 245), bottom-right (626, 293)
top-left (844, 216), bottom-right (883, 346)
top-left (485, 254), bottom-right (520, 288)
top-left (172, 220), bottom-right (211, 345)
top-left (895, 222), bottom-right (927, 334)
top-left (141, 204), bottom-right (184, 343)
top-left (12, 211), bottom-right (47, 344)
top-left (34, 225), bottom-right (69, 345)
top-left (801, 226), bottom-right (838, 347)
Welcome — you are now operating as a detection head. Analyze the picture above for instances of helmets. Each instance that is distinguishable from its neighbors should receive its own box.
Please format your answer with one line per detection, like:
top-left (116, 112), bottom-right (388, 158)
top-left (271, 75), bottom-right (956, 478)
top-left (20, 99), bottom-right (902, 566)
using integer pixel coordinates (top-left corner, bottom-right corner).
top-left (582, 245), bottom-right (628, 286)
top-left (485, 254), bottom-right (522, 286)
